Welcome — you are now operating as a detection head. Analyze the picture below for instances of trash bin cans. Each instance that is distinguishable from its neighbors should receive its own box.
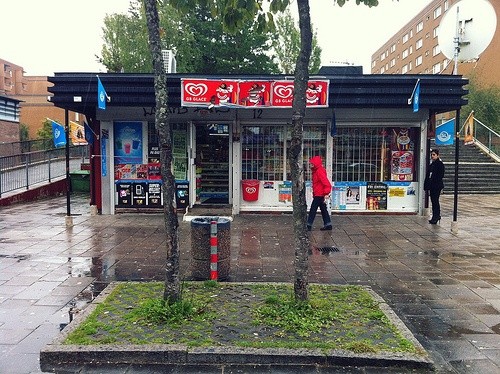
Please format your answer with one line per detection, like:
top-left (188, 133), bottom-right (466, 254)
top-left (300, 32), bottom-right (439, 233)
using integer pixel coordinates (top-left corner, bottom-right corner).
top-left (69, 169), bottom-right (90, 192)
top-left (191, 218), bottom-right (231, 281)
top-left (240, 179), bottom-right (260, 201)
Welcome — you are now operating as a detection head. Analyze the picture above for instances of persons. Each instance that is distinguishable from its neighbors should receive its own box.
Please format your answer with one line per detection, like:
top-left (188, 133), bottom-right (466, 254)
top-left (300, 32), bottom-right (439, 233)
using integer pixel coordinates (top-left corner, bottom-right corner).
top-left (424, 150), bottom-right (445, 224)
top-left (307, 156), bottom-right (333, 231)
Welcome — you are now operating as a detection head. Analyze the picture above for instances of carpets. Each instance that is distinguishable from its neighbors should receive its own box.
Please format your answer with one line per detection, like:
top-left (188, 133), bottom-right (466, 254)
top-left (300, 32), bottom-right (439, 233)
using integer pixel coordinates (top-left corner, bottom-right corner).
top-left (201, 197), bottom-right (228, 204)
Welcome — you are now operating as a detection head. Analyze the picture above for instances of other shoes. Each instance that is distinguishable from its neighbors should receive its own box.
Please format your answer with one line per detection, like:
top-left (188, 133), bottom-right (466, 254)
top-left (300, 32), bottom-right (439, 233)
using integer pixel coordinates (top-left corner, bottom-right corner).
top-left (320, 225), bottom-right (332, 230)
top-left (307, 225), bottom-right (311, 231)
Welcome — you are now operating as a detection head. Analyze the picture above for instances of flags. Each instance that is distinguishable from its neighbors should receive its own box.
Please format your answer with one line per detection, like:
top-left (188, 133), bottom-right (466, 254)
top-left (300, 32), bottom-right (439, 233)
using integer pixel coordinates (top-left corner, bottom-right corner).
top-left (464, 114), bottom-right (473, 145)
top-left (435, 119), bottom-right (454, 145)
top-left (97, 77), bottom-right (105, 109)
top-left (413, 83), bottom-right (419, 112)
top-left (51, 121), bottom-right (66, 148)
top-left (84, 122), bottom-right (95, 145)
top-left (71, 122), bottom-right (88, 145)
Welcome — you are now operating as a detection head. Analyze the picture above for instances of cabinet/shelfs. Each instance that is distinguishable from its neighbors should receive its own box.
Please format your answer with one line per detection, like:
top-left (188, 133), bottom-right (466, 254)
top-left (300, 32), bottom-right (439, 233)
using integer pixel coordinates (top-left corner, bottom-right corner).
top-left (200, 161), bottom-right (229, 194)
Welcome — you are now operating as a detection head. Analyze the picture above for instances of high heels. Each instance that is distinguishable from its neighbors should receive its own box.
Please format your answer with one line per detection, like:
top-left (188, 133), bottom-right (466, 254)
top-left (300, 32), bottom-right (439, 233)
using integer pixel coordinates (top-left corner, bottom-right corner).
top-left (429, 216), bottom-right (441, 224)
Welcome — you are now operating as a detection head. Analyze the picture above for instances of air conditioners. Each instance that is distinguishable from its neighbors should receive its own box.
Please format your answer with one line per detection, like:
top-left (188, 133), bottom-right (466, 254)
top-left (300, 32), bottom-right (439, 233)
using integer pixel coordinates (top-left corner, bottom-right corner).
top-left (161, 50), bottom-right (177, 74)
